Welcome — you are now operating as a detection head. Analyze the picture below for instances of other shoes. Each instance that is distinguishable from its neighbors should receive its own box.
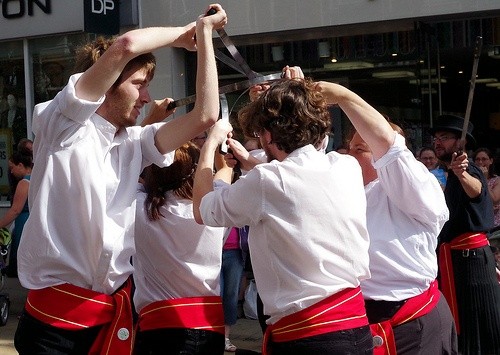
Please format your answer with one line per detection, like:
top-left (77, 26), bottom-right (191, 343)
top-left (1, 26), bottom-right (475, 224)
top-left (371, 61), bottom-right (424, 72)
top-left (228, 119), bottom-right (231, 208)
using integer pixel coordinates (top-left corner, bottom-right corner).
top-left (224, 338), bottom-right (238, 352)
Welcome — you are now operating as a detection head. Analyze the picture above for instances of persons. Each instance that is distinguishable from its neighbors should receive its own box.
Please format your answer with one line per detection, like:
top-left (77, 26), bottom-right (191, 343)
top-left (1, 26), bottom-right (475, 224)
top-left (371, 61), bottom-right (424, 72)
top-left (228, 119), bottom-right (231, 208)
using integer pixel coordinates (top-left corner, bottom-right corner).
top-left (14, 4), bottom-right (227, 355)
top-left (0, 64), bottom-right (500, 355)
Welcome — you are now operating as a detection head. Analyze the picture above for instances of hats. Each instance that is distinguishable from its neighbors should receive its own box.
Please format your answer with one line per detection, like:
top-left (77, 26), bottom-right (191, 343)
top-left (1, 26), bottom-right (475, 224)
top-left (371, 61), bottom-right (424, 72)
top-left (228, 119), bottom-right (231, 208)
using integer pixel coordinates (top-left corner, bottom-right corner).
top-left (428, 114), bottom-right (474, 147)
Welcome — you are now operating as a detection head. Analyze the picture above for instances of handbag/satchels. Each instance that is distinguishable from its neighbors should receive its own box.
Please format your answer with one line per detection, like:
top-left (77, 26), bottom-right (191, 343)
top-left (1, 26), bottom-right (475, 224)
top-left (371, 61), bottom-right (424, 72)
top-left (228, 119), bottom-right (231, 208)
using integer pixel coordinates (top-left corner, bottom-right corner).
top-left (243, 279), bottom-right (258, 320)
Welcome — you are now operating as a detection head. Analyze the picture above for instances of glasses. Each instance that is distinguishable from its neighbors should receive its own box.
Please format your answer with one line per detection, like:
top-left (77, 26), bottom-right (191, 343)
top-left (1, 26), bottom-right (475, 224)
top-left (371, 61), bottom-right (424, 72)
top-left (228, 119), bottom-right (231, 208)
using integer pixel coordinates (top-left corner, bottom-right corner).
top-left (251, 127), bottom-right (264, 138)
top-left (431, 135), bottom-right (455, 143)
top-left (474, 157), bottom-right (490, 162)
